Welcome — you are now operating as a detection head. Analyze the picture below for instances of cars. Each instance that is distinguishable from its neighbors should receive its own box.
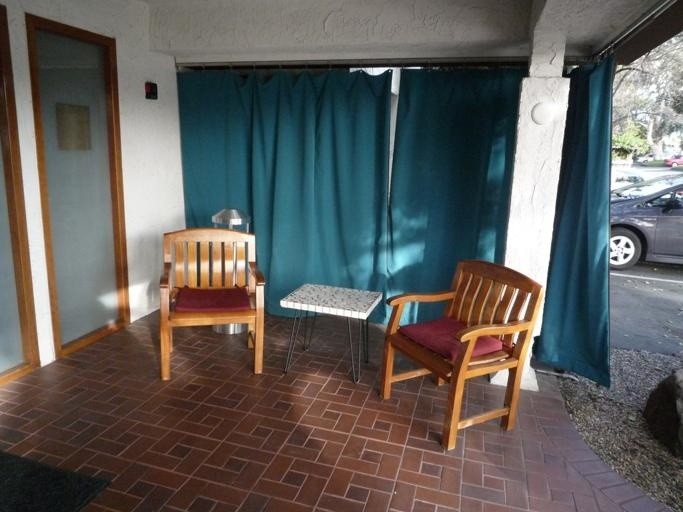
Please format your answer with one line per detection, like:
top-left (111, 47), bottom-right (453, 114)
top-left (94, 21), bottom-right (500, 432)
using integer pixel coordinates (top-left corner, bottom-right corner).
top-left (610, 172), bottom-right (682, 271)
top-left (636, 154), bottom-right (683, 167)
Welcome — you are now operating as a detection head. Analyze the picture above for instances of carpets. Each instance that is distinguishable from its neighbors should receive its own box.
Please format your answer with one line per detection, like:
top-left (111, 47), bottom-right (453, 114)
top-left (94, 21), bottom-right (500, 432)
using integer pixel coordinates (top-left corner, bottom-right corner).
top-left (0, 449), bottom-right (113, 511)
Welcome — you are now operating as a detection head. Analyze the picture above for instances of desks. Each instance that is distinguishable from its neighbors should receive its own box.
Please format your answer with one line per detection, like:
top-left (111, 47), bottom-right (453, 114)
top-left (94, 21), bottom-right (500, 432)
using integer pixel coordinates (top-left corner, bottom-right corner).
top-left (278, 284), bottom-right (383, 386)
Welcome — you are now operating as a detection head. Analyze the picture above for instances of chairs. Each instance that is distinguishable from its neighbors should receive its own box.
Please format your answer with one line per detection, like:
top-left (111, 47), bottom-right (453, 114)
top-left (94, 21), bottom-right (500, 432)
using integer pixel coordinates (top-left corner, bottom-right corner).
top-left (377, 257), bottom-right (545, 450)
top-left (158, 225), bottom-right (266, 380)
